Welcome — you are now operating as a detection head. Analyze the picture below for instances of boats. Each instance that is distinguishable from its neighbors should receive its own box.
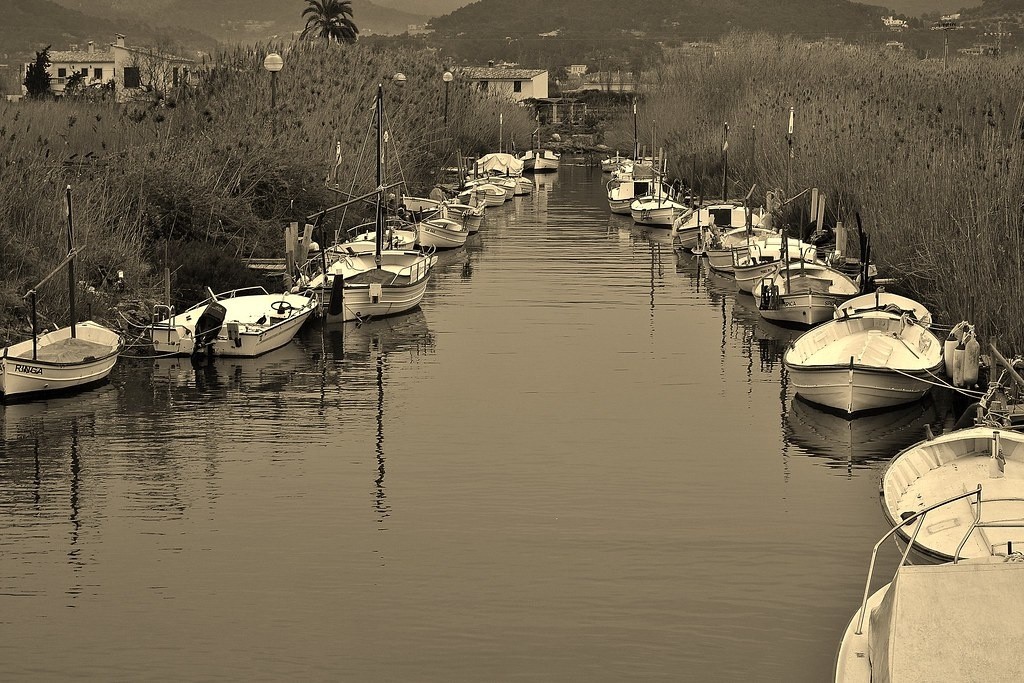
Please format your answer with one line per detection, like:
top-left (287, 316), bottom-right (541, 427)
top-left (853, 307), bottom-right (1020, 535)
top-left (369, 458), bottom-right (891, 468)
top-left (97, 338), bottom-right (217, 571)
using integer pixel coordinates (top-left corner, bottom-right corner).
top-left (600, 96), bottom-right (1023, 683)
top-left (0, 83), bottom-right (562, 403)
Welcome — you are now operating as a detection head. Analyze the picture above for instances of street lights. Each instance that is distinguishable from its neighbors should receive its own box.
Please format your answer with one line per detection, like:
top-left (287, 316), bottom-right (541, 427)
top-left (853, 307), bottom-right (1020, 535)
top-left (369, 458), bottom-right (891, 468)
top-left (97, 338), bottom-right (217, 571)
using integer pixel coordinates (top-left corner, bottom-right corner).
top-left (442, 71), bottom-right (454, 137)
top-left (264, 52), bottom-right (285, 150)
